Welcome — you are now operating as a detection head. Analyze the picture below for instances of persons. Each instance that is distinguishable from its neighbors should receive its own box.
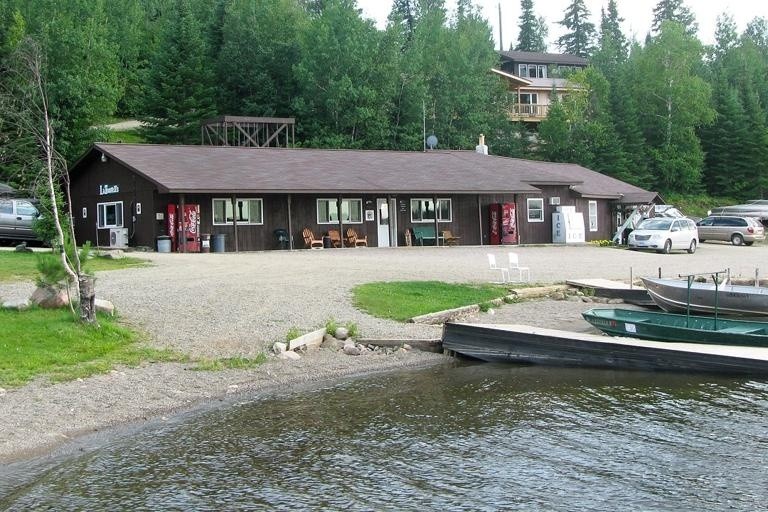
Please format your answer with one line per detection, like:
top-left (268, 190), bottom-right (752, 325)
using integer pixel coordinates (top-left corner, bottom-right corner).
top-left (624, 213), bottom-right (636, 245)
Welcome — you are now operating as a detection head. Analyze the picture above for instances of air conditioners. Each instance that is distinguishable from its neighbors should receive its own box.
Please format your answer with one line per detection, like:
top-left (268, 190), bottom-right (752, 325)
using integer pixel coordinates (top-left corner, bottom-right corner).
top-left (110, 227), bottom-right (128, 248)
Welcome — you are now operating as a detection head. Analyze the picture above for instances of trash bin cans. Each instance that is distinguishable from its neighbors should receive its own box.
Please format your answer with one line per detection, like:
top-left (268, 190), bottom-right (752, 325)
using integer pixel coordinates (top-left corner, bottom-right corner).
top-left (273, 229), bottom-right (289, 250)
top-left (156, 235), bottom-right (172, 253)
top-left (199, 233), bottom-right (227, 253)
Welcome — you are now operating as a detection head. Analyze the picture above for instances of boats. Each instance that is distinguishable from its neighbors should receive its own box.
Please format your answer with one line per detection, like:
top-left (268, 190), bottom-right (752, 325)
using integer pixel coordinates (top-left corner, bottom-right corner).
top-left (639, 275), bottom-right (768, 318)
top-left (580, 307), bottom-right (768, 348)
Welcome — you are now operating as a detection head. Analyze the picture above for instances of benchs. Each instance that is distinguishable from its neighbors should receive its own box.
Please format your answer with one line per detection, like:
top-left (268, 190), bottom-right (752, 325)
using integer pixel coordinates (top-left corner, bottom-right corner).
top-left (412, 226), bottom-right (444, 245)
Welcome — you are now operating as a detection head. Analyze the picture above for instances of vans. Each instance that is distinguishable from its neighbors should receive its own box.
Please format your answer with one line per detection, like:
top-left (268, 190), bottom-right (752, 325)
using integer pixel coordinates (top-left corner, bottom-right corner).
top-left (695, 215), bottom-right (766, 246)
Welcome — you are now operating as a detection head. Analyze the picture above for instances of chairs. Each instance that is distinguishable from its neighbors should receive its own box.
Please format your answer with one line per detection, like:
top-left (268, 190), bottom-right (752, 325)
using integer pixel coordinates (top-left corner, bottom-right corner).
top-left (273, 227), bottom-right (368, 248)
top-left (442, 230), bottom-right (460, 245)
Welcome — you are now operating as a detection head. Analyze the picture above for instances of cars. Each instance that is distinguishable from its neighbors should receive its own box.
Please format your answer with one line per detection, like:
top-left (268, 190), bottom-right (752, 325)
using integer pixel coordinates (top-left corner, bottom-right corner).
top-left (0, 197), bottom-right (47, 242)
top-left (626, 216), bottom-right (699, 254)
